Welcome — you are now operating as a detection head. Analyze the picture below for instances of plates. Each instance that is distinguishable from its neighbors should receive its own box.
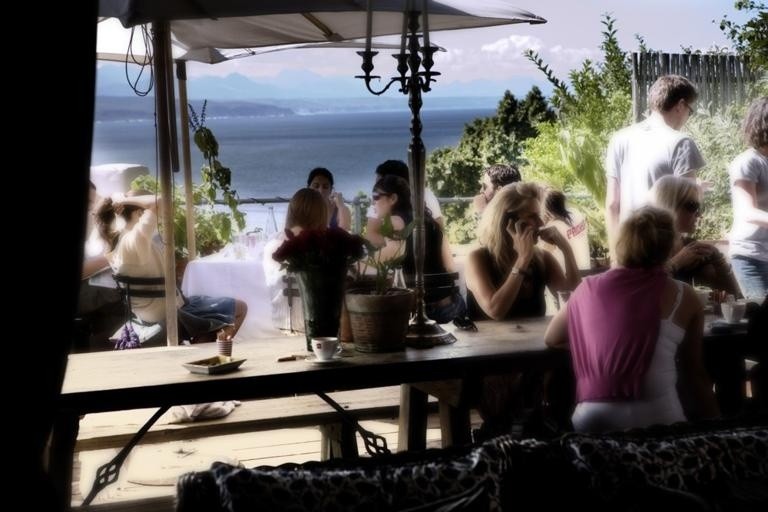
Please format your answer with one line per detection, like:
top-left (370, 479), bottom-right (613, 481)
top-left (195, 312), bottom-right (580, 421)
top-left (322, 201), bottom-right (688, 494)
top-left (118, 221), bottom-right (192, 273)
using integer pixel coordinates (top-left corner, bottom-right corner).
top-left (183, 354), bottom-right (248, 377)
top-left (305, 354), bottom-right (342, 365)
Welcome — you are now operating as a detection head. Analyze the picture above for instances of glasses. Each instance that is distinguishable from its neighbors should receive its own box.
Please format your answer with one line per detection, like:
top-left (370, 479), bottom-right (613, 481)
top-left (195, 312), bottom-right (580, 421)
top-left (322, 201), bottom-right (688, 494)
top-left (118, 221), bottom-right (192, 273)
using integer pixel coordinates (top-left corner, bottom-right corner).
top-left (371, 191), bottom-right (392, 200)
top-left (683, 101), bottom-right (694, 116)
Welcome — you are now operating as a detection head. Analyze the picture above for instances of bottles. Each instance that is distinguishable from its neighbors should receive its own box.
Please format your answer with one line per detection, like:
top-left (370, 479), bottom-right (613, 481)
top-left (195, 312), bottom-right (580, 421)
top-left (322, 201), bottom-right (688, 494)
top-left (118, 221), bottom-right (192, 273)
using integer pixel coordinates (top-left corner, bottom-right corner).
top-left (266, 205), bottom-right (277, 237)
top-left (391, 265), bottom-right (408, 289)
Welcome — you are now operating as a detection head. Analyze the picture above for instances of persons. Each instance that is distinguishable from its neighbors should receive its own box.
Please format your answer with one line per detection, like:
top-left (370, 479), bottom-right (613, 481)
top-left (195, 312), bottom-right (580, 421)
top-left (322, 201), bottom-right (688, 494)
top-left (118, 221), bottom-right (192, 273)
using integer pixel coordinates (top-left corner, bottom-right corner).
top-left (465, 182), bottom-right (580, 427)
top-left (729, 95), bottom-right (768, 306)
top-left (305, 166), bottom-right (350, 235)
top-left (266, 188), bottom-right (330, 243)
top-left (546, 207), bottom-right (709, 433)
top-left (75, 179), bottom-right (126, 317)
top-left (643, 176), bottom-right (745, 421)
top-left (95, 188), bottom-right (247, 342)
top-left (365, 162), bottom-right (444, 245)
top-left (472, 166), bottom-right (520, 214)
top-left (604, 74), bottom-right (715, 272)
top-left (535, 181), bottom-right (592, 316)
top-left (364, 175), bottom-right (466, 324)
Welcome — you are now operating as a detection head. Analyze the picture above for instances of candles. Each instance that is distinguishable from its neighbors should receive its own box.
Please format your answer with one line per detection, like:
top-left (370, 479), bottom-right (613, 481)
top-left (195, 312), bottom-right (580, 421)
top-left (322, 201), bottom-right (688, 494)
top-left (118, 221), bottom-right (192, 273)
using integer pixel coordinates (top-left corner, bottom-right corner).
top-left (363, 0), bottom-right (373, 52)
top-left (399, 0), bottom-right (408, 54)
top-left (421, 0), bottom-right (429, 45)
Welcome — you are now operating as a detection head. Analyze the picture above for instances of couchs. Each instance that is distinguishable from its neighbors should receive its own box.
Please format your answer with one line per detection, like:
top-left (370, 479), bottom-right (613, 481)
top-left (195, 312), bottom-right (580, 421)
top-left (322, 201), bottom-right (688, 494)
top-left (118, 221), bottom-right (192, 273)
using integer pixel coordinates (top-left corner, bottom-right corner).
top-left (165, 421), bottom-right (765, 511)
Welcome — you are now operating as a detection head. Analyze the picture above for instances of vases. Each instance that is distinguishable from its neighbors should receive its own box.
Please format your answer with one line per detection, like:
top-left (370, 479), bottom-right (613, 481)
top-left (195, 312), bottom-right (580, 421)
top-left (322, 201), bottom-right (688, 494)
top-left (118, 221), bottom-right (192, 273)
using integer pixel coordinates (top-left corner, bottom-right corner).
top-left (290, 257), bottom-right (347, 353)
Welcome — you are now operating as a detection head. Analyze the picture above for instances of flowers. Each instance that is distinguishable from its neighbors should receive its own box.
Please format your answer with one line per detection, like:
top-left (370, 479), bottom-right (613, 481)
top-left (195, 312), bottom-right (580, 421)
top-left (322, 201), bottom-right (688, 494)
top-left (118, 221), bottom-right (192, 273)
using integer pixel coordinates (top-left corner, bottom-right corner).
top-left (269, 221), bottom-right (366, 280)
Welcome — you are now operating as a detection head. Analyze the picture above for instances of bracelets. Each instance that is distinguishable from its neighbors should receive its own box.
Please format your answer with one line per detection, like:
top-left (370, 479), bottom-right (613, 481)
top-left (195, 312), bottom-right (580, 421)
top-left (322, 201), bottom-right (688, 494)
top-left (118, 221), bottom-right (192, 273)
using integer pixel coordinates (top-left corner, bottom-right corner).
top-left (511, 268), bottom-right (527, 274)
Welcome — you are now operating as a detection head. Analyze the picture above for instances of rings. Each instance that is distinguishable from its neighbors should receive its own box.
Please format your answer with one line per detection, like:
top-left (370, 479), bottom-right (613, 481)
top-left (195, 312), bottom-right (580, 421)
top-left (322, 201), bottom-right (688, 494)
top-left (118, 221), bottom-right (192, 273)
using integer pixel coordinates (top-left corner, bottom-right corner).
top-left (696, 248), bottom-right (697, 251)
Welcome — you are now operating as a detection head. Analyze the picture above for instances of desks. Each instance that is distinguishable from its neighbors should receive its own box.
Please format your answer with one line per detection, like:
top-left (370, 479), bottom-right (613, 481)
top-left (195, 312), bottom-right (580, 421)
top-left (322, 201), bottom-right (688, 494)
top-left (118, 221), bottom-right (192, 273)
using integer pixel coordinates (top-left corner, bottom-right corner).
top-left (57, 311), bottom-right (766, 511)
top-left (180, 238), bottom-right (291, 341)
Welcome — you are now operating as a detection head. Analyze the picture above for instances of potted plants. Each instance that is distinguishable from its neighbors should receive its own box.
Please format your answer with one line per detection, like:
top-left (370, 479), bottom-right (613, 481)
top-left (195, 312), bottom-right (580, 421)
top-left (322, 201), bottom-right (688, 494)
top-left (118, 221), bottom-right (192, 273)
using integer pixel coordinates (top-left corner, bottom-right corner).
top-left (343, 213), bottom-right (425, 356)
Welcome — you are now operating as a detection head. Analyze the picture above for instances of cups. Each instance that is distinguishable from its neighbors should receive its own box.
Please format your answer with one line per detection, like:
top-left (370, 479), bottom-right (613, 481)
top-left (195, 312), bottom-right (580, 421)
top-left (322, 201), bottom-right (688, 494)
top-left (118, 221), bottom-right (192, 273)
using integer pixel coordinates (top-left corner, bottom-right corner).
top-left (216, 340), bottom-right (231, 357)
top-left (231, 232), bottom-right (246, 259)
top-left (721, 302), bottom-right (747, 322)
top-left (311, 337), bottom-right (343, 359)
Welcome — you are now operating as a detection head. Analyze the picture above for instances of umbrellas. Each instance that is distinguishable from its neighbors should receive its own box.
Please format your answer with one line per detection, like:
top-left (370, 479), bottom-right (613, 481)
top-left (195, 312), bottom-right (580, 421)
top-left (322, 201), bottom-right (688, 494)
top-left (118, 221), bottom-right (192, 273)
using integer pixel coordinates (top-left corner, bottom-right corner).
top-left (94, 0), bottom-right (549, 345)
top-left (171, 31), bottom-right (448, 262)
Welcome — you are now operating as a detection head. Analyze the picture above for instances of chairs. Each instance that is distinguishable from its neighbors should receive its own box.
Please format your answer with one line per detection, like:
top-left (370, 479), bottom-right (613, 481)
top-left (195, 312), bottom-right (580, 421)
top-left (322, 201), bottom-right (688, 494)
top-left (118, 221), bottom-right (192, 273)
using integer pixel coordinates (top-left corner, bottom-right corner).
top-left (402, 267), bottom-right (461, 318)
top-left (106, 270), bottom-right (204, 345)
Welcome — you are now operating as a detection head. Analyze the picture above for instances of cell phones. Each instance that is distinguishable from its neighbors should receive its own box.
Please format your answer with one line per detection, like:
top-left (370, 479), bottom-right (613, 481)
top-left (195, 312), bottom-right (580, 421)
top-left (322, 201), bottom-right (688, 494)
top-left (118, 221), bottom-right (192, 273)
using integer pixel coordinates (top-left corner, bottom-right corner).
top-left (504, 213), bottom-right (519, 225)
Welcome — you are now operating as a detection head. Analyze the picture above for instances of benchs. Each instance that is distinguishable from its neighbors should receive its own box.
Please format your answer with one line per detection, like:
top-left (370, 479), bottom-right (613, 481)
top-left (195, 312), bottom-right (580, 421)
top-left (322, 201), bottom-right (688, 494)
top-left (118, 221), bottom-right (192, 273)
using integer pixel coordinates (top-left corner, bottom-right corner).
top-left (70, 386), bottom-right (445, 490)
